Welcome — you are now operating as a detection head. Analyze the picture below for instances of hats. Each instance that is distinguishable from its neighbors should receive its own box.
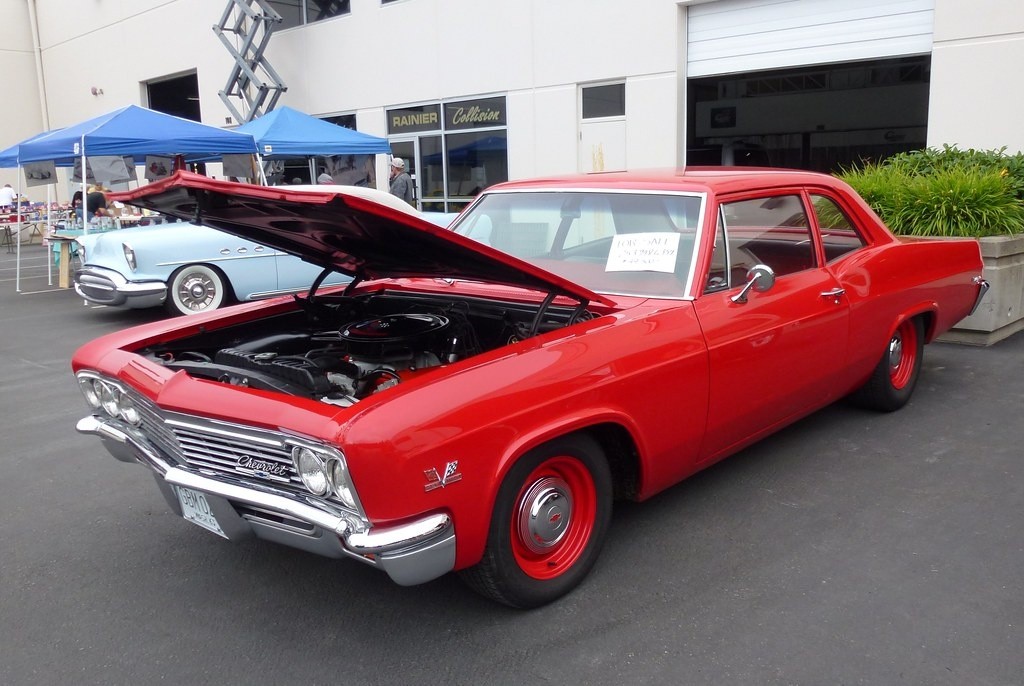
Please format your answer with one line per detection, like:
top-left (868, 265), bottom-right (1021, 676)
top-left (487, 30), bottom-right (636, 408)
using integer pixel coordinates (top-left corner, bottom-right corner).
top-left (389, 157), bottom-right (405, 168)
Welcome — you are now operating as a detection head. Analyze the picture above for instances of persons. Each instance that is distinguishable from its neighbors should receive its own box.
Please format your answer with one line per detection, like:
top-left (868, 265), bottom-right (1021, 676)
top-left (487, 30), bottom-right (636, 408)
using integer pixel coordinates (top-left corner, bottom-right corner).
top-left (76, 179), bottom-right (113, 223)
top-left (293, 178), bottom-right (303, 185)
top-left (390, 158), bottom-right (414, 207)
top-left (1, 184), bottom-right (18, 214)
top-left (317, 165), bottom-right (335, 183)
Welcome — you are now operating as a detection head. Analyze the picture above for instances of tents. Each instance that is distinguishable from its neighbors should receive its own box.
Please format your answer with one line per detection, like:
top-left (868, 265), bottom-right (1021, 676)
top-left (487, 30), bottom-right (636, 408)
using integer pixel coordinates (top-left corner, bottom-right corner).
top-left (0, 104), bottom-right (257, 306)
top-left (228, 106), bottom-right (394, 187)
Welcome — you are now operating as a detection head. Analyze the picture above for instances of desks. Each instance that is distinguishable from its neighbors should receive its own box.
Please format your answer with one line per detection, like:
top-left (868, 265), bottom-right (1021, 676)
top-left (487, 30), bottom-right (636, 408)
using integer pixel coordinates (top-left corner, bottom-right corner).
top-left (0, 210), bottom-right (164, 282)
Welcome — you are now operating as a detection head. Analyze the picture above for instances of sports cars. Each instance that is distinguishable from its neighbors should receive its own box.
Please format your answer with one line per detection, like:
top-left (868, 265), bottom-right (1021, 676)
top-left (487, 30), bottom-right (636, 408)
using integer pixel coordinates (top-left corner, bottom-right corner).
top-left (70, 166), bottom-right (990, 612)
top-left (68, 184), bottom-right (461, 319)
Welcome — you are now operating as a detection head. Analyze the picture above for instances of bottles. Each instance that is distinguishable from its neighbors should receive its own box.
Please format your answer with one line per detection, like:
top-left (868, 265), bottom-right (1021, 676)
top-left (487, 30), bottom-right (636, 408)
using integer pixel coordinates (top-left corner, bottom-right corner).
top-left (116, 217), bottom-right (121, 229)
top-left (35, 212), bottom-right (39, 221)
top-left (65, 216), bottom-right (82, 230)
